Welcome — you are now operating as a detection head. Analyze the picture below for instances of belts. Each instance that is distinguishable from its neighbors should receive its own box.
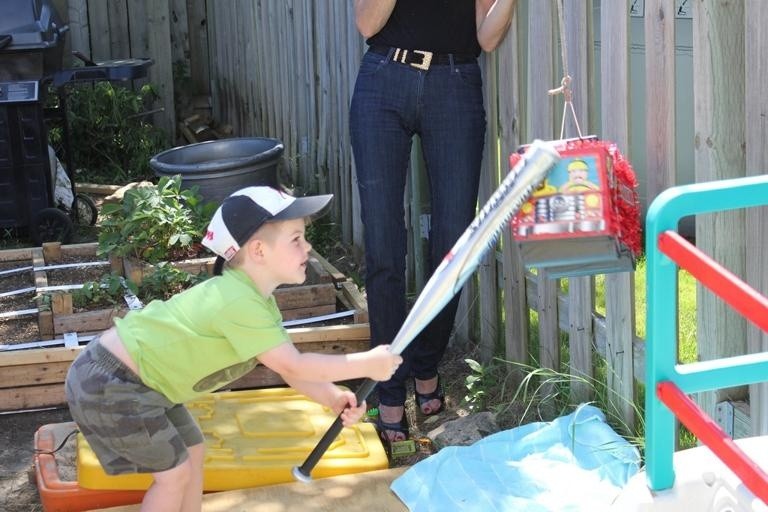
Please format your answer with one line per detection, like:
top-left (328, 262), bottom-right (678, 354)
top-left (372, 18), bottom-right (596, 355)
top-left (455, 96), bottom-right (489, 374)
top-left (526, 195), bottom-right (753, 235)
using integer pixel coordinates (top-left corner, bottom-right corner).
top-left (366, 43), bottom-right (478, 71)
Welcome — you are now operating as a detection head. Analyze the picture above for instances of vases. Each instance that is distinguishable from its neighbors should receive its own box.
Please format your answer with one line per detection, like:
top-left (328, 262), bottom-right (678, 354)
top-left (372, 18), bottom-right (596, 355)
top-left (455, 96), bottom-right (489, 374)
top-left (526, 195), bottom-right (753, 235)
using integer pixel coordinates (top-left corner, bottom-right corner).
top-left (150, 137), bottom-right (285, 206)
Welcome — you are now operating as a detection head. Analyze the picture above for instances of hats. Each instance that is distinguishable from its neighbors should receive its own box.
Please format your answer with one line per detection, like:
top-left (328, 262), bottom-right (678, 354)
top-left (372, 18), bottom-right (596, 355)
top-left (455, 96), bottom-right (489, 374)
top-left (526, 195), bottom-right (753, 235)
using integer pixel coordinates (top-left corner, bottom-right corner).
top-left (200, 185), bottom-right (334, 262)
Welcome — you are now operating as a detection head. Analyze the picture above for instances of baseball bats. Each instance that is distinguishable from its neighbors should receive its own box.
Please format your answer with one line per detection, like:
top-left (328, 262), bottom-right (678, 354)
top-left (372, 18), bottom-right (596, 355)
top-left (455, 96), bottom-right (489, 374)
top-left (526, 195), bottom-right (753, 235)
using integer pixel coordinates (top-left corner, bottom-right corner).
top-left (292, 135), bottom-right (560, 483)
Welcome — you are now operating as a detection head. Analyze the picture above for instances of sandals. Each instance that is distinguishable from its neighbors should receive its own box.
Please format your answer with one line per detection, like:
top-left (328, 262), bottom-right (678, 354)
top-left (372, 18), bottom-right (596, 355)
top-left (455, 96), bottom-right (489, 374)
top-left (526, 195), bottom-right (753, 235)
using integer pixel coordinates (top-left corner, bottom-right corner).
top-left (415, 374), bottom-right (445, 416)
top-left (377, 406), bottom-right (409, 446)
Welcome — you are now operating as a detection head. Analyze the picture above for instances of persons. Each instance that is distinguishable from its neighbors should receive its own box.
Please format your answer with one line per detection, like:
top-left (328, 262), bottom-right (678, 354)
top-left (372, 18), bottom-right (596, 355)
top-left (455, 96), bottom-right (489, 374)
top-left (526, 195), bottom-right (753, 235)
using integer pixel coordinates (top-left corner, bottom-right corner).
top-left (64, 183), bottom-right (405, 512)
top-left (349, 0), bottom-right (513, 443)
